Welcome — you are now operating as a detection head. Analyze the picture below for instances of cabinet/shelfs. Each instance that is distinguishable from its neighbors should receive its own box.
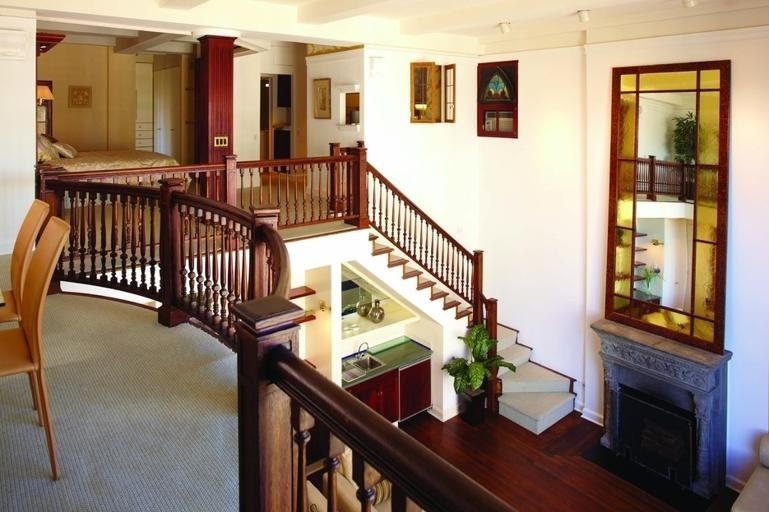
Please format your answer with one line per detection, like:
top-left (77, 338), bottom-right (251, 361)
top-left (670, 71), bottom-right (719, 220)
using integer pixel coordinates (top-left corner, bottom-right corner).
top-left (399, 357), bottom-right (431, 422)
top-left (633, 231), bottom-right (661, 314)
top-left (135, 54), bottom-right (195, 165)
top-left (344, 367), bottom-right (398, 431)
top-left (287, 287), bottom-right (317, 325)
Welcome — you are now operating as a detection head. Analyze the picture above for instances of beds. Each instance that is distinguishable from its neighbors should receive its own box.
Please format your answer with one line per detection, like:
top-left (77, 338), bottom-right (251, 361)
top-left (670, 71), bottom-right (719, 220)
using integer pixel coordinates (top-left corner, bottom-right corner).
top-left (40, 148), bottom-right (191, 212)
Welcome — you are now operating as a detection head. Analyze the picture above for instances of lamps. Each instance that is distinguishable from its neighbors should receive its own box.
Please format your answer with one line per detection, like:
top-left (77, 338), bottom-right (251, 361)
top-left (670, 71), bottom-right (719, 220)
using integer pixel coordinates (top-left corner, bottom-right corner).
top-left (37, 86), bottom-right (55, 106)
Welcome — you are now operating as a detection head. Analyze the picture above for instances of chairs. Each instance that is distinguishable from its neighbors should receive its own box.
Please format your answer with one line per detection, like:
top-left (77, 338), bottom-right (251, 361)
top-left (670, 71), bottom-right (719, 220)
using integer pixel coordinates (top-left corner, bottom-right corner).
top-left (598, 60), bottom-right (733, 355)
top-left (1, 199), bottom-right (72, 481)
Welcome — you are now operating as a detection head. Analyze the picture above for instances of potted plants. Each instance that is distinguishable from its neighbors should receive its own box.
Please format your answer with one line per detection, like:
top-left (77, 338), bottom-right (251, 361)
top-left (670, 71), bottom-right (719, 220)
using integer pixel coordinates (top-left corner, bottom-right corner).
top-left (442, 326), bottom-right (518, 426)
top-left (672, 112), bottom-right (699, 200)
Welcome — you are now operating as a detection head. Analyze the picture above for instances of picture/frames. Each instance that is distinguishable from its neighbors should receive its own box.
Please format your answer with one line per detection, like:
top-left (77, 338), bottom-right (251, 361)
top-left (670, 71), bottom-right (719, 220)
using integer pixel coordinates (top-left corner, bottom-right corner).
top-left (69, 85), bottom-right (92, 109)
top-left (312, 79), bottom-right (332, 118)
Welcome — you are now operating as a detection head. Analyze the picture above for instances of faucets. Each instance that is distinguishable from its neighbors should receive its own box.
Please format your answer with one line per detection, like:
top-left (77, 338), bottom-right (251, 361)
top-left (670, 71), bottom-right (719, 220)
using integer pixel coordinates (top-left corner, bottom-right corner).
top-left (356, 341), bottom-right (370, 359)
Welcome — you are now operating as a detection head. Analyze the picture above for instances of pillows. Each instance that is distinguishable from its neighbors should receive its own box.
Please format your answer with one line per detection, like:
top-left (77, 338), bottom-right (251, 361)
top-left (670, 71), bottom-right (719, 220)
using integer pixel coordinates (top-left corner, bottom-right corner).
top-left (38, 133), bottom-right (79, 163)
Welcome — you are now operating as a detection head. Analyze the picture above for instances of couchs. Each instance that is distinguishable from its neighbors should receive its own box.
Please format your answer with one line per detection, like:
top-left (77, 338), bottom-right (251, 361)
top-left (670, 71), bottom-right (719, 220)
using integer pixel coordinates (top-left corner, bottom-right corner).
top-left (307, 447), bottom-right (426, 512)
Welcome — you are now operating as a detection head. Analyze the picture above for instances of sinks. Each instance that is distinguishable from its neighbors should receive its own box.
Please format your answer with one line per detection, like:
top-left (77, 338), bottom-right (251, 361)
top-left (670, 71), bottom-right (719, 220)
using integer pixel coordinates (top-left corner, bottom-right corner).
top-left (353, 355), bottom-right (383, 373)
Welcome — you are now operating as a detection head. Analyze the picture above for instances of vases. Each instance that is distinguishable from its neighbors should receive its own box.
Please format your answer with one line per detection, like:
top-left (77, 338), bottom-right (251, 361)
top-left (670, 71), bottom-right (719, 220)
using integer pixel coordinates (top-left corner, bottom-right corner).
top-left (368, 299), bottom-right (384, 323)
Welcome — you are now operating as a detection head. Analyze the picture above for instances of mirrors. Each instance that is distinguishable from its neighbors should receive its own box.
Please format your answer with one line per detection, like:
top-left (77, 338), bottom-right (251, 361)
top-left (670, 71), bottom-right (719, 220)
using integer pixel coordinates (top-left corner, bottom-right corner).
top-left (336, 85), bottom-right (361, 133)
